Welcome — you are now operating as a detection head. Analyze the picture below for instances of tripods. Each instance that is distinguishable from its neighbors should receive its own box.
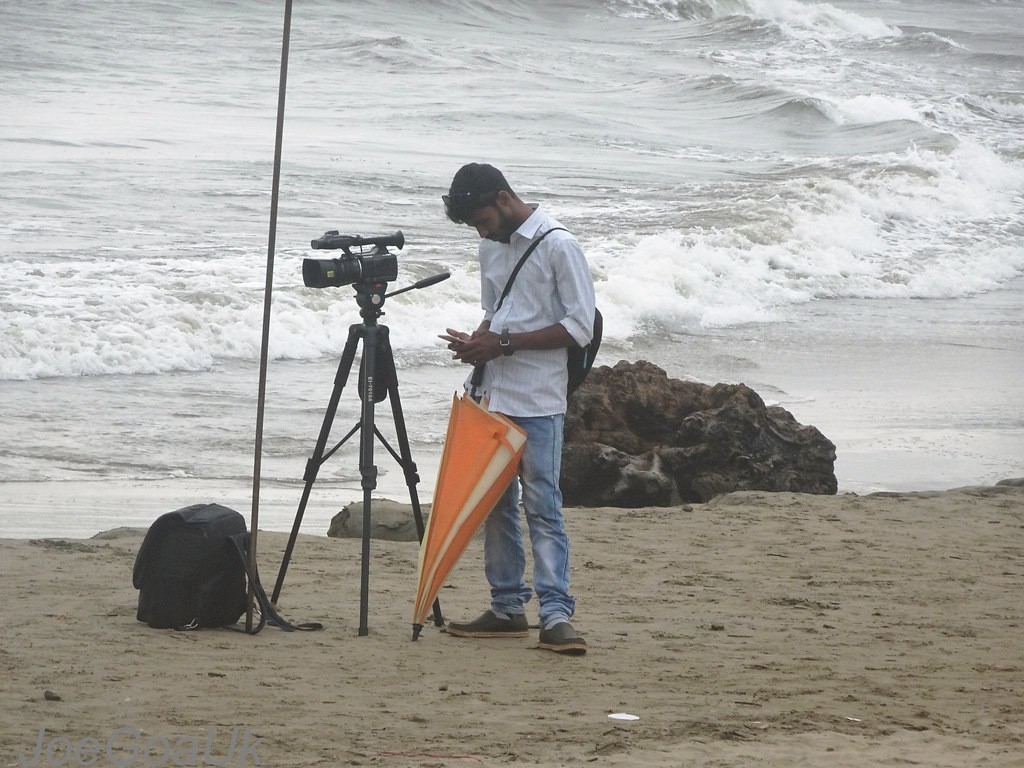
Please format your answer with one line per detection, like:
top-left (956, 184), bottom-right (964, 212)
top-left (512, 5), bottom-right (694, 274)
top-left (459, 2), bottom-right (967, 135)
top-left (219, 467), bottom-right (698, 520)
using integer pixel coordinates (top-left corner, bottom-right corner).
top-left (271, 271), bottom-right (449, 637)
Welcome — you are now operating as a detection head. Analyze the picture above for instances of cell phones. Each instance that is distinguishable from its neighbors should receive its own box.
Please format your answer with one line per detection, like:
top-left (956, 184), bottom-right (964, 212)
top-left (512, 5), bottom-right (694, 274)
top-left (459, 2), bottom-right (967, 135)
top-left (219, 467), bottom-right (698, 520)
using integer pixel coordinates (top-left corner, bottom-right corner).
top-left (438, 336), bottom-right (468, 344)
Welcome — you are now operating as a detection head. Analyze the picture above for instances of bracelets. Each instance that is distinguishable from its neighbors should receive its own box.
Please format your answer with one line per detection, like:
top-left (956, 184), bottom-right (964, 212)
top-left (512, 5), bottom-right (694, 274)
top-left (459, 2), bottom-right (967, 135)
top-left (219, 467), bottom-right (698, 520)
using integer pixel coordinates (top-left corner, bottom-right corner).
top-left (500, 328), bottom-right (513, 356)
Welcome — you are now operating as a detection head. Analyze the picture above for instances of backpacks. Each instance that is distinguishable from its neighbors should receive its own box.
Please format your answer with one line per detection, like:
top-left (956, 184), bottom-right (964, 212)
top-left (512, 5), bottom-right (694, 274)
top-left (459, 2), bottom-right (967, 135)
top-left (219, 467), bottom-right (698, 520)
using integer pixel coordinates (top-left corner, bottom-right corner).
top-left (132, 502), bottom-right (323, 635)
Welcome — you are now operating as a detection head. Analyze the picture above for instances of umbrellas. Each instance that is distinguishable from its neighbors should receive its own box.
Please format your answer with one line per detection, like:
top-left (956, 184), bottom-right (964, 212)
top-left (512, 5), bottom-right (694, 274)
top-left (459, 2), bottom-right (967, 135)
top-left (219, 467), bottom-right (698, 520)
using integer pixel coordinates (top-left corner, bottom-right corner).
top-left (413, 363), bottom-right (527, 640)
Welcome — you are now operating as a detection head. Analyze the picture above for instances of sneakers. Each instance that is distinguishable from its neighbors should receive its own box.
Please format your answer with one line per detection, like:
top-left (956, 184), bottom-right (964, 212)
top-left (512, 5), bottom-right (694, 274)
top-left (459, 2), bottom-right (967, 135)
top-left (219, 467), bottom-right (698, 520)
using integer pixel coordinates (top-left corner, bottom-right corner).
top-left (538, 622), bottom-right (588, 653)
top-left (446, 610), bottom-right (530, 638)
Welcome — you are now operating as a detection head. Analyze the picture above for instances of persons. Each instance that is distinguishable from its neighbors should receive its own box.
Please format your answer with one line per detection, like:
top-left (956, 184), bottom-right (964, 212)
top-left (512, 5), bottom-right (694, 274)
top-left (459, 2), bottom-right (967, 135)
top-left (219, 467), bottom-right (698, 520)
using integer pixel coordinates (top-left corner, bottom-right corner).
top-left (444, 162), bottom-right (596, 655)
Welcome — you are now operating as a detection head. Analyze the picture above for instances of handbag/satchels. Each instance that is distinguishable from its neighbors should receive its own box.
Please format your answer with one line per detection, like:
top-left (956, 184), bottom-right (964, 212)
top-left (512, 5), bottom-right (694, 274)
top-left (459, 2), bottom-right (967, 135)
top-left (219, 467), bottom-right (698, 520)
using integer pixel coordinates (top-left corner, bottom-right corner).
top-left (567, 305), bottom-right (603, 398)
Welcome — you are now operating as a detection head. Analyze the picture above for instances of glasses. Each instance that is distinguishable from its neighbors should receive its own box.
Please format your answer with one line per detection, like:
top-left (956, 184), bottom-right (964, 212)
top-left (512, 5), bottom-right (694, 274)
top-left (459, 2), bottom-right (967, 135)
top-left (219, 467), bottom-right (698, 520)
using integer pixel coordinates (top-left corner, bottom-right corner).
top-left (442, 191), bottom-right (489, 208)
